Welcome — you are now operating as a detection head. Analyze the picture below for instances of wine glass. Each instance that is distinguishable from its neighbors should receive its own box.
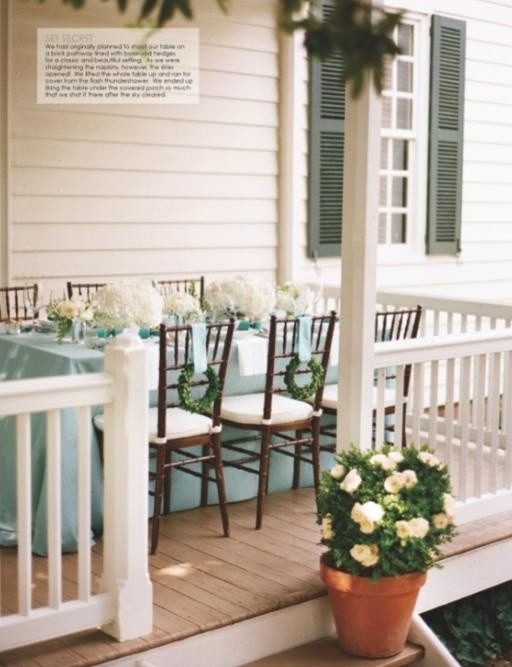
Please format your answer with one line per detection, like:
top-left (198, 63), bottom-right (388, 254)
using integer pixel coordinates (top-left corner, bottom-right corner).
top-left (25, 284), bottom-right (44, 336)
top-left (49, 289), bottom-right (69, 340)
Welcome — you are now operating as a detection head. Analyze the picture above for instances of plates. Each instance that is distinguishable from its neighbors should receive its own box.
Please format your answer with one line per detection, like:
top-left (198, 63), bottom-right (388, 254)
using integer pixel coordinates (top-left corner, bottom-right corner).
top-left (208, 330), bottom-right (258, 340)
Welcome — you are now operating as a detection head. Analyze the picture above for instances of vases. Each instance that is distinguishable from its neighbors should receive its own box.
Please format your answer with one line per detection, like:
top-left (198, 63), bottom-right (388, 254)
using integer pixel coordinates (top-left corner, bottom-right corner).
top-left (319, 552), bottom-right (427, 659)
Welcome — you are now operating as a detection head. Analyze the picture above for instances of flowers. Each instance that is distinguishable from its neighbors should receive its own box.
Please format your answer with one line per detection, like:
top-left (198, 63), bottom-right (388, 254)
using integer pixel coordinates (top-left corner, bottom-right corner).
top-left (204, 291), bottom-right (236, 315)
top-left (161, 294), bottom-right (200, 314)
top-left (314, 442), bottom-right (459, 587)
top-left (275, 283), bottom-right (326, 317)
top-left (45, 287), bottom-right (153, 345)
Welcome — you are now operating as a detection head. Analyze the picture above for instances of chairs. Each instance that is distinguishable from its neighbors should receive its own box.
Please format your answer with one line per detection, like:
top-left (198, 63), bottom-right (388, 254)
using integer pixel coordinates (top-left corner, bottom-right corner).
top-left (0, 282), bottom-right (39, 323)
top-left (152, 275), bottom-right (205, 313)
top-left (93, 318), bottom-right (235, 554)
top-left (371, 306), bottom-right (423, 447)
top-left (67, 281), bottom-right (108, 302)
top-left (200, 310), bottom-right (336, 528)
